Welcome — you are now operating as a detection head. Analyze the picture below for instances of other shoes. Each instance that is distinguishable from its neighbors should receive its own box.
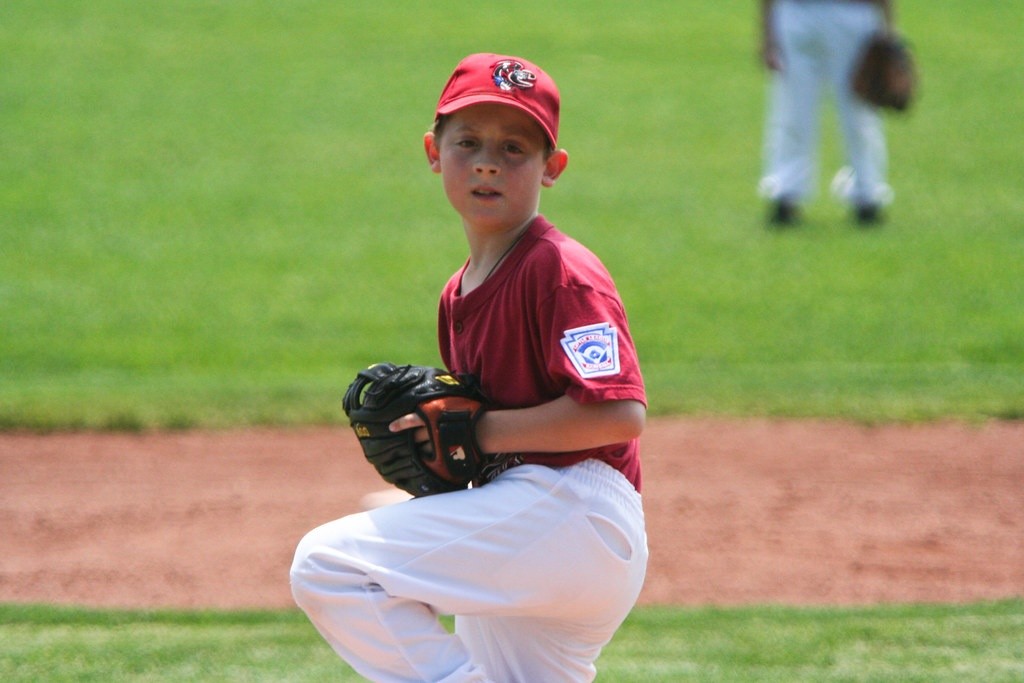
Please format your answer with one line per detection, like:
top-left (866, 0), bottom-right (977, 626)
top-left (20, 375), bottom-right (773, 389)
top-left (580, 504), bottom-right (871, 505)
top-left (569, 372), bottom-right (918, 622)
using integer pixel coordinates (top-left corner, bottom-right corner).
top-left (856, 207), bottom-right (885, 228)
top-left (767, 200), bottom-right (797, 228)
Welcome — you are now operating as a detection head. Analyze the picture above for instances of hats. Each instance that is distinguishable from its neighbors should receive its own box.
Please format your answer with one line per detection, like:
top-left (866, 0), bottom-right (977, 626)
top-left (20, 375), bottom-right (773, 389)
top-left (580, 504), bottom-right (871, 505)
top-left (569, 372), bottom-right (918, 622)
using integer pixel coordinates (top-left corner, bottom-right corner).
top-left (435, 53), bottom-right (560, 151)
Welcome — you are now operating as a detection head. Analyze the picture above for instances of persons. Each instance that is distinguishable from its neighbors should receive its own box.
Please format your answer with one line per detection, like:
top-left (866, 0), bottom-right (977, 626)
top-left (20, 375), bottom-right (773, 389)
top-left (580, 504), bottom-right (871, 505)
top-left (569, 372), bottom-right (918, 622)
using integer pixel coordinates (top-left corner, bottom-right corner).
top-left (758, 0), bottom-right (916, 231)
top-left (288, 54), bottom-right (647, 683)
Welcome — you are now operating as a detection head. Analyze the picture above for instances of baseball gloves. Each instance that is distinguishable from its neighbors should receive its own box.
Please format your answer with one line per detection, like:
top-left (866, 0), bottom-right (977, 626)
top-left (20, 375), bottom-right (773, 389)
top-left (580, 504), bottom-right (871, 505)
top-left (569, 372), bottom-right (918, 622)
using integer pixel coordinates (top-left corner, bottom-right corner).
top-left (852, 31), bottom-right (916, 113)
top-left (341, 359), bottom-right (519, 499)
